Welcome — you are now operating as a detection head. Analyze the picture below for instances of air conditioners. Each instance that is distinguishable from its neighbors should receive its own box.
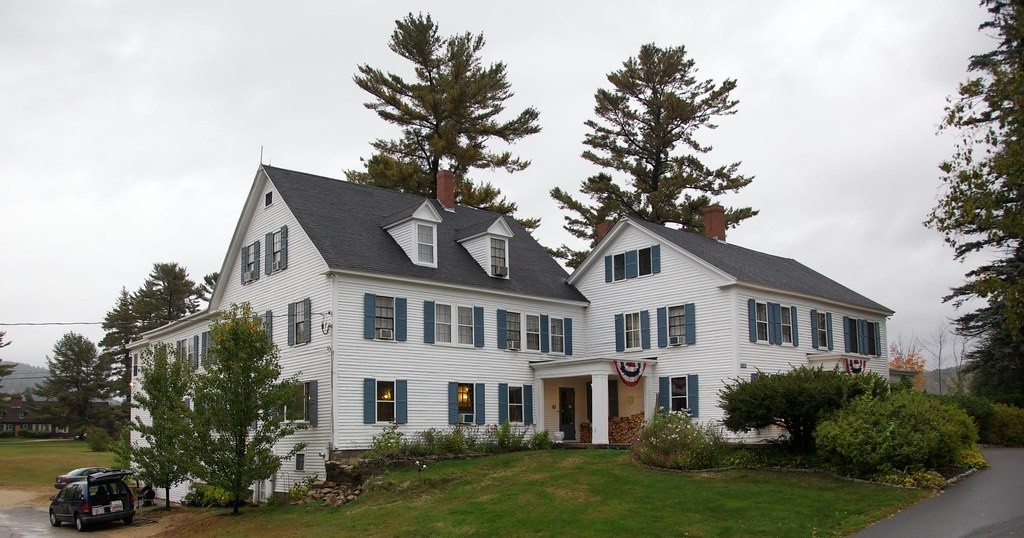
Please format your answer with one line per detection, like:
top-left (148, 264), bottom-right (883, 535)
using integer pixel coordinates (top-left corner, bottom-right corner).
top-left (459, 414), bottom-right (475, 424)
top-left (244, 271), bottom-right (254, 282)
top-left (507, 340), bottom-right (520, 350)
top-left (495, 266), bottom-right (507, 276)
top-left (669, 336), bottom-right (684, 346)
top-left (272, 261), bottom-right (280, 271)
top-left (376, 329), bottom-right (394, 340)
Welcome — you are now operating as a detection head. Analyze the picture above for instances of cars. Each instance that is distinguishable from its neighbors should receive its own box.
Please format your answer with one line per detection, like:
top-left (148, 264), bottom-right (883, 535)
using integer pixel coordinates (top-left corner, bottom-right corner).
top-left (49, 469), bottom-right (136, 532)
top-left (55, 467), bottom-right (109, 489)
